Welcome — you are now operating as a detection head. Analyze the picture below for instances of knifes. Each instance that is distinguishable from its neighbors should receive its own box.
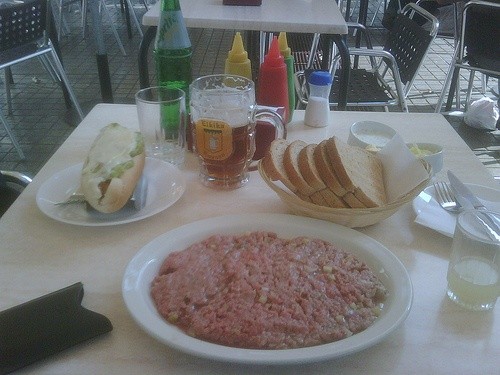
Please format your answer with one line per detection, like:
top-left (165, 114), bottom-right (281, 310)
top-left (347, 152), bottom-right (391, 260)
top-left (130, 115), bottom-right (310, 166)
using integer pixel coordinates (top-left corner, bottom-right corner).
top-left (446, 169), bottom-right (500, 235)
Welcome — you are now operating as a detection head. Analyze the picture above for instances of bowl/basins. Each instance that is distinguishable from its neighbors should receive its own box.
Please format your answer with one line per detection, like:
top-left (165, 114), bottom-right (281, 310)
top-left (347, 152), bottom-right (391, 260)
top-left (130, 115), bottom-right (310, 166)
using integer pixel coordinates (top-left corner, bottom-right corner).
top-left (407, 142), bottom-right (444, 178)
top-left (347, 120), bottom-right (401, 154)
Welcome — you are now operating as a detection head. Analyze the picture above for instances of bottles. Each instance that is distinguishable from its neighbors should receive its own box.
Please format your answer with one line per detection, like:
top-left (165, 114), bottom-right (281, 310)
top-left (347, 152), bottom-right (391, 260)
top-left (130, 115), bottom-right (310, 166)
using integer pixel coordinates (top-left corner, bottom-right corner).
top-left (153, 0), bottom-right (194, 141)
top-left (303, 72), bottom-right (333, 128)
top-left (223, 31), bottom-right (295, 125)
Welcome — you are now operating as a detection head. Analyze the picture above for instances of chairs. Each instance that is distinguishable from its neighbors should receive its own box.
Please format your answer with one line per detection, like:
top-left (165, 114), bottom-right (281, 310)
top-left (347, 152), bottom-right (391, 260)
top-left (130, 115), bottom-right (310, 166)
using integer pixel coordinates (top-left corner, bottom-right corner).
top-left (0, 0), bottom-right (500, 220)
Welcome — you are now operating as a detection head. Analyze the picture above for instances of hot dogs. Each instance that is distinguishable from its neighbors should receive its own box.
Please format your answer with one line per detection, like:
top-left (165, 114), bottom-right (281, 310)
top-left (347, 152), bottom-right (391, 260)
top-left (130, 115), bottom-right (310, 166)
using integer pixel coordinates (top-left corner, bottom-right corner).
top-left (80, 123), bottom-right (145, 213)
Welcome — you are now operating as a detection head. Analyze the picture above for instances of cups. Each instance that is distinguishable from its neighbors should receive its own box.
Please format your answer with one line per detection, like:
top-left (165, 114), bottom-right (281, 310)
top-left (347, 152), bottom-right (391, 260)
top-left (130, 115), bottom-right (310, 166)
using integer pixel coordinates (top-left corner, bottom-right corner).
top-left (444, 211), bottom-right (500, 311)
top-left (189, 73), bottom-right (289, 191)
top-left (135, 86), bottom-right (187, 167)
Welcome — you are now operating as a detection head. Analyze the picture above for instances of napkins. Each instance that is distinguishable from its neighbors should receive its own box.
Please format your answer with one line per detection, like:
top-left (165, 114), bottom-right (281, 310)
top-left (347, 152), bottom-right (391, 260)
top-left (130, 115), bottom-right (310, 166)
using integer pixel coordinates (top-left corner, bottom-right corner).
top-left (0, 282), bottom-right (113, 375)
top-left (414, 191), bottom-right (500, 240)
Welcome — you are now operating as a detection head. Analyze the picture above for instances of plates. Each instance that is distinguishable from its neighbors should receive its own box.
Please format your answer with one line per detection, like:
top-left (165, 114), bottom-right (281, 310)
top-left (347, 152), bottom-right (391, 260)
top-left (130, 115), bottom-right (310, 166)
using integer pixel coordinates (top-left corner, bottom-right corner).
top-left (35, 156), bottom-right (188, 227)
top-left (120, 212), bottom-right (414, 364)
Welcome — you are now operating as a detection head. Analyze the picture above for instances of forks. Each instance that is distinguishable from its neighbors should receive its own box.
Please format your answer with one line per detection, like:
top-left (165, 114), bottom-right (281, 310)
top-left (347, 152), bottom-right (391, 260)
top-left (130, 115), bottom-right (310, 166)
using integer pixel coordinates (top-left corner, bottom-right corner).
top-left (432, 182), bottom-right (500, 247)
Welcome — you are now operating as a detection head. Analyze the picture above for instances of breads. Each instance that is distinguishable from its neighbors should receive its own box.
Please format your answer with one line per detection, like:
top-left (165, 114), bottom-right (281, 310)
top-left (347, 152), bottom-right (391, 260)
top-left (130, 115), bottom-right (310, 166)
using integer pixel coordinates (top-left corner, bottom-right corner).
top-left (263, 136), bottom-right (388, 208)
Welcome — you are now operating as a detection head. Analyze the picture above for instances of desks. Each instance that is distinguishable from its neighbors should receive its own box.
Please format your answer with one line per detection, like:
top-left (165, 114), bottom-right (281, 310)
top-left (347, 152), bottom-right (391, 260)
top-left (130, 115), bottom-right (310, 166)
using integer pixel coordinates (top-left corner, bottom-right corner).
top-left (138, 0), bottom-right (351, 111)
top-left (0, 100), bottom-right (500, 375)
